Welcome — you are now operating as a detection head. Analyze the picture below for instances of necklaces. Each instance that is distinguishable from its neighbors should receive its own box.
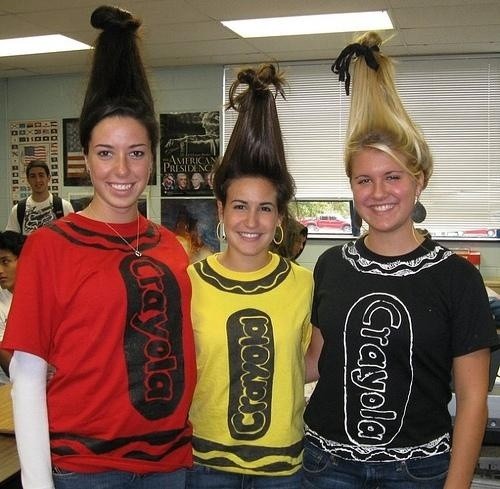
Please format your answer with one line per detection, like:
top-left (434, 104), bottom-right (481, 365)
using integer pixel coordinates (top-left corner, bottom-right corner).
top-left (85, 200), bottom-right (150, 257)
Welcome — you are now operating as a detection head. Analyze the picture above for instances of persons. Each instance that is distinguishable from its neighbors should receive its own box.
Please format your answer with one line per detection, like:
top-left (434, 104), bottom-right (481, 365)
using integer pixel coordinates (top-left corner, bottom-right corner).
top-left (5, 161), bottom-right (75, 235)
top-left (272, 216), bottom-right (308, 262)
top-left (302, 31), bottom-right (499, 488)
top-left (162, 172), bottom-right (214, 193)
top-left (0, 230), bottom-right (34, 384)
top-left (1, 5), bottom-right (197, 489)
top-left (187, 62), bottom-right (315, 488)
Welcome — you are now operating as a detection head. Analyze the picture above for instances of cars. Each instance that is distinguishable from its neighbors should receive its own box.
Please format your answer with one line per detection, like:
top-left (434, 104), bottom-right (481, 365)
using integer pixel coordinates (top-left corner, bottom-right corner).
top-left (451, 228), bottom-right (496, 237)
top-left (301, 212), bottom-right (351, 231)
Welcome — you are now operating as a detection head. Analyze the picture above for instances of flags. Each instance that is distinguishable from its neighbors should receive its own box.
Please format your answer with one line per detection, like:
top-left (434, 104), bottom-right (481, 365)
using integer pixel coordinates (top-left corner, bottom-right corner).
top-left (10, 121), bottom-right (58, 198)
